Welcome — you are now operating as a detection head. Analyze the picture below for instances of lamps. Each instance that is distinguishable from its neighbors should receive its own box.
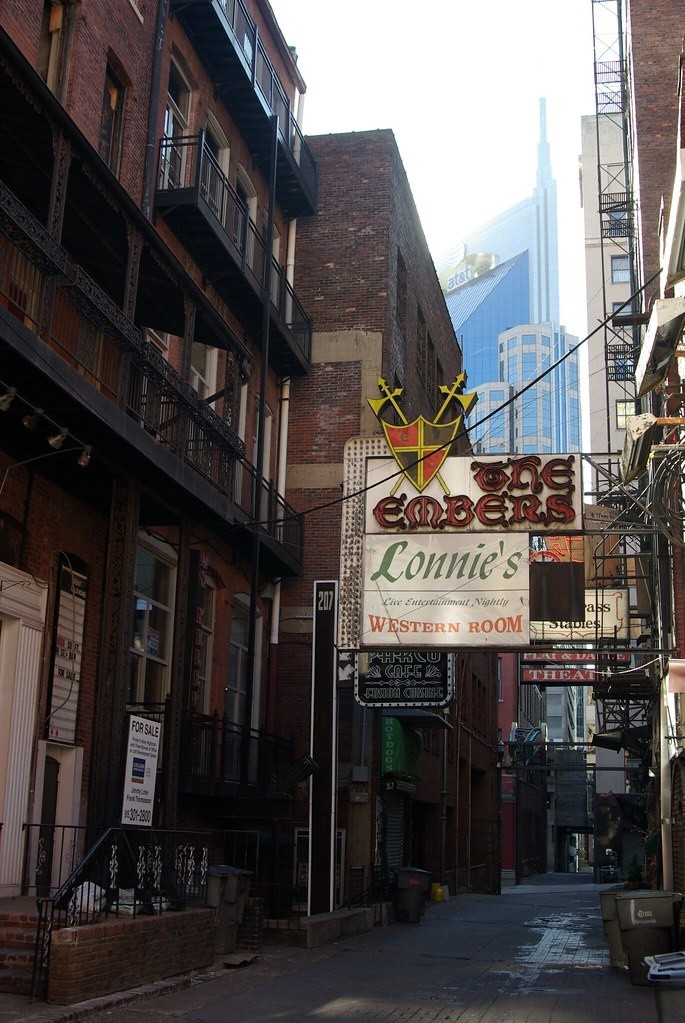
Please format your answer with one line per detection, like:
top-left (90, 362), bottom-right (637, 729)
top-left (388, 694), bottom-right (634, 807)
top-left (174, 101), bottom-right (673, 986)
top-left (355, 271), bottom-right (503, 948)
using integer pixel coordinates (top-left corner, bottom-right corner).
top-left (0, 379), bottom-right (92, 467)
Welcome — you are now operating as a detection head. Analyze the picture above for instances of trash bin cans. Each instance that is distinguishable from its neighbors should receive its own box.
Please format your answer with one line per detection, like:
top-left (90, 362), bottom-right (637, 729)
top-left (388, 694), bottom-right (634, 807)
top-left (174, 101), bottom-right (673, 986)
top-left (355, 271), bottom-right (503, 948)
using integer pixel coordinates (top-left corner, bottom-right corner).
top-left (640, 950), bottom-right (685, 1023)
top-left (392, 865), bottom-right (430, 926)
top-left (617, 891), bottom-right (682, 986)
top-left (206, 864), bottom-right (254, 954)
top-left (598, 889), bottom-right (650, 969)
